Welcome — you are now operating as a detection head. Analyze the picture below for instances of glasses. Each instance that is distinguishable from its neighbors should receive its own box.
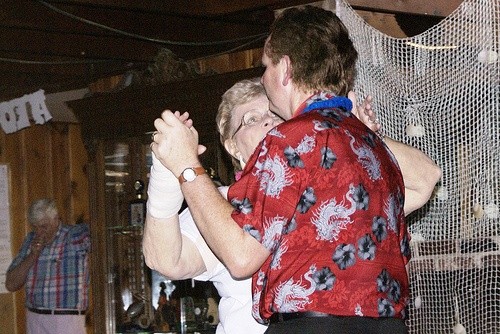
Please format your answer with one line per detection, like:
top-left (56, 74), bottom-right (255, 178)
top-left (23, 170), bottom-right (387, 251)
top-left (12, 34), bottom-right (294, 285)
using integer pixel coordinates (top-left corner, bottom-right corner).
top-left (230, 108), bottom-right (282, 140)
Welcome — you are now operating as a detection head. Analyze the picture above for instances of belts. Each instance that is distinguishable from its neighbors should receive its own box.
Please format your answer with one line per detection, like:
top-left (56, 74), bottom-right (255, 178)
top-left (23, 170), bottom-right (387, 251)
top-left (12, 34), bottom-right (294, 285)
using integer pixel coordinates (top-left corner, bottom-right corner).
top-left (266, 309), bottom-right (340, 322)
top-left (27, 305), bottom-right (86, 314)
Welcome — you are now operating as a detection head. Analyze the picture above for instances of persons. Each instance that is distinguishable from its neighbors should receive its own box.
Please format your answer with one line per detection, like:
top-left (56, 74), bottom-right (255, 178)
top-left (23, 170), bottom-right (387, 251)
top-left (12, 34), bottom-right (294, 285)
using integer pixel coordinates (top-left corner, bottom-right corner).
top-left (150, 5), bottom-right (411, 334)
top-left (5, 199), bottom-right (91, 334)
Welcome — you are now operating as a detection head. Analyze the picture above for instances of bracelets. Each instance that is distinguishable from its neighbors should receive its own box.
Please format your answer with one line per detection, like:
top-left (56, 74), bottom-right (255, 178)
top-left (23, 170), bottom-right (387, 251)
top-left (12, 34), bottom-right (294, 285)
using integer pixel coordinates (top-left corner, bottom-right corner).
top-left (375, 131), bottom-right (385, 141)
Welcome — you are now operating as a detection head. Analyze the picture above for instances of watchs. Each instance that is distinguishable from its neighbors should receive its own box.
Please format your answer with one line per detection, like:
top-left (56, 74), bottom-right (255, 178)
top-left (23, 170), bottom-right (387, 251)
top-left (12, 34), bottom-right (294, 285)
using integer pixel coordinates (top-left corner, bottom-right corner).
top-left (178, 167), bottom-right (207, 185)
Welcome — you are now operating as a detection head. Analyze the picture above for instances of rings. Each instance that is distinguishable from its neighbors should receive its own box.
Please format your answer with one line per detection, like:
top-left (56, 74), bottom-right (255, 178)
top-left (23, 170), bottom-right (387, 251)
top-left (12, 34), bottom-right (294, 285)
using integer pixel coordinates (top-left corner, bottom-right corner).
top-left (368, 118), bottom-right (379, 123)
top-left (142, 79), bottom-right (442, 334)
top-left (150, 133), bottom-right (158, 151)
